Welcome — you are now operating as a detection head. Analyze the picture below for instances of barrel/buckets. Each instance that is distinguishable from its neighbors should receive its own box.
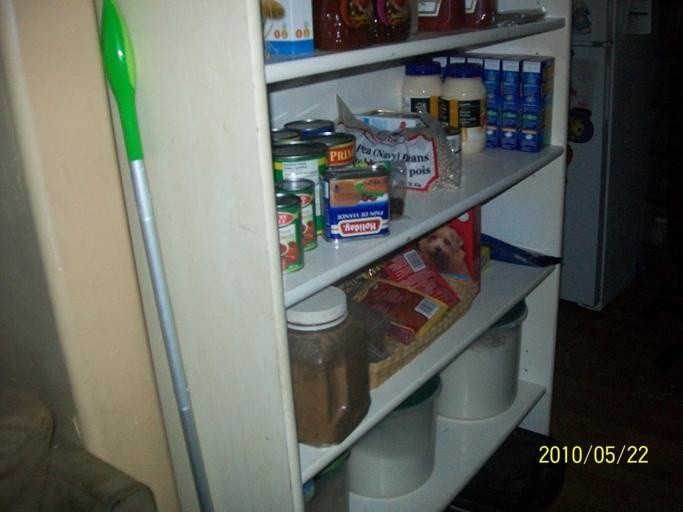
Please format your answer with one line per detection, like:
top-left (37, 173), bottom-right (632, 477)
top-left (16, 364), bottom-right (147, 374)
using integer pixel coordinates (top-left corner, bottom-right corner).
top-left (438, 304), bottom-right (528, 421)
top-left (349, 375), bottom-right (442, 499)
top-left (300, 452), bottom-right (349, 512)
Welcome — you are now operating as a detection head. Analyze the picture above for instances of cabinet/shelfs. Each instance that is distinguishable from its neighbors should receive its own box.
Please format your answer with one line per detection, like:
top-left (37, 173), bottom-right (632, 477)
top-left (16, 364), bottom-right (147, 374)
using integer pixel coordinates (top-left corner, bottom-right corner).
top-left (94, 1), bottom-right (572, 512)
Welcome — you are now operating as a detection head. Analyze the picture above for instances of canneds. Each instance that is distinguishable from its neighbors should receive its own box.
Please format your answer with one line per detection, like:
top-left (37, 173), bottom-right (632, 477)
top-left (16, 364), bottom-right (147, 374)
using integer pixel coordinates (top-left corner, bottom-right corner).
top-left (271, 62), bottom-right (487, 276)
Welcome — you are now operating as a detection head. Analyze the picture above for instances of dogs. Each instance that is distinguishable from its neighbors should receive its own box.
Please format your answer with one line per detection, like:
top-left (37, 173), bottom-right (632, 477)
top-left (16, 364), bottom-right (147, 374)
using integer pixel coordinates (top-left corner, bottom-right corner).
top-left (418, 225), bottom-right (469, 275)
